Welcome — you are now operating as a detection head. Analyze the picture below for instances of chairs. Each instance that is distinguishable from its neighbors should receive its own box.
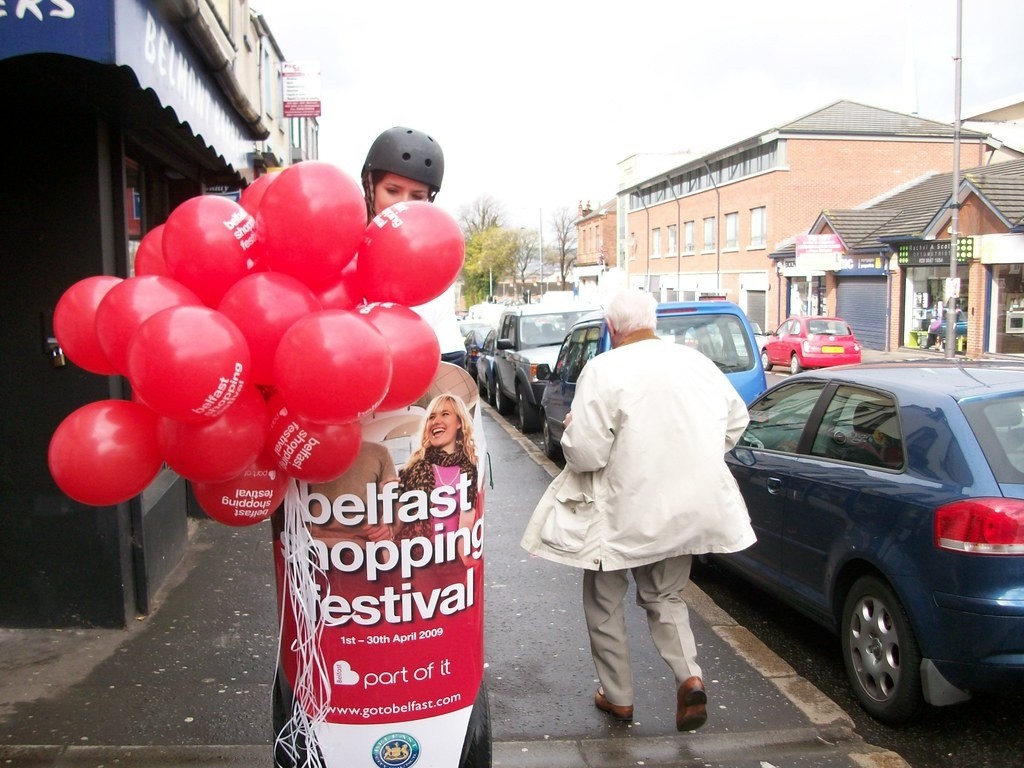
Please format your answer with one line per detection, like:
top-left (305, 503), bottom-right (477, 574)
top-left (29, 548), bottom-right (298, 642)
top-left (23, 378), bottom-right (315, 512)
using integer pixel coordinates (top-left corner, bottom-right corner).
top-left (853, 401), bottom-right (897, 433)
top-left (522, 322), bottom-right (539, 337)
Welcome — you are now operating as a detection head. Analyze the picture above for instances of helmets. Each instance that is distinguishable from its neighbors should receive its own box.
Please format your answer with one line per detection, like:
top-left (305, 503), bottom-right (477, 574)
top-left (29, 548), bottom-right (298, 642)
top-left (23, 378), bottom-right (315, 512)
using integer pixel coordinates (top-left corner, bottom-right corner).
top-left (361, 126), bottom-right (444, 192)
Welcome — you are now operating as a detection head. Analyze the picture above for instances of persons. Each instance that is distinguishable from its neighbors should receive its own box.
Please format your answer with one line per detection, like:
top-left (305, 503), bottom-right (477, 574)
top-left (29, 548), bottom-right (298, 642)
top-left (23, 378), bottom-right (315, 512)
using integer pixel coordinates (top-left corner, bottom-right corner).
top-left (304, 442), bottom-right (399, 544)
top-left (396, 393), bottom-right (480, 567)
top-left (520, 288), bottom-right (758, 731)
top-left (360, 129), bottom-right (445, 222)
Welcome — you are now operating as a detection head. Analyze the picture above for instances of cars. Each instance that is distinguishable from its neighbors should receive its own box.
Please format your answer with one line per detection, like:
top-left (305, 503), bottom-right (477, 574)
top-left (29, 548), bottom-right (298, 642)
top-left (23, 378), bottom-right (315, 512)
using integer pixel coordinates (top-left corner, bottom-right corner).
top-left (477, 328), bottom-right (499, 404)
top-left (465, 328), bottom-right (491, 378)
top-left (460, 321), bottom-right (487, 341)
top-left (760, 316), bottom-right (861, 374)
top-left (692, 360), bottom-right (1024, 722)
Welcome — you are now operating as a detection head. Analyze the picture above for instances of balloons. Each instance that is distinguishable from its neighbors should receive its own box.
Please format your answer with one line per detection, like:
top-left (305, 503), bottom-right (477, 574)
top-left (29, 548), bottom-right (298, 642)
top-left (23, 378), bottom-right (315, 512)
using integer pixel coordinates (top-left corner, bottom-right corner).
top-left (47, 160), bottom-right (467, 528)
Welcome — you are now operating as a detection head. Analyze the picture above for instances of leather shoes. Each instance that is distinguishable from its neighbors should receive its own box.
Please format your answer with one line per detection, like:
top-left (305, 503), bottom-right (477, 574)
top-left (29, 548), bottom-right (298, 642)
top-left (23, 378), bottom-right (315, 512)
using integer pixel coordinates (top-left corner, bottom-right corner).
top-left (676, 675), bottom-right (708, 732)
top-left (595, 686), bottom-right (634, 721)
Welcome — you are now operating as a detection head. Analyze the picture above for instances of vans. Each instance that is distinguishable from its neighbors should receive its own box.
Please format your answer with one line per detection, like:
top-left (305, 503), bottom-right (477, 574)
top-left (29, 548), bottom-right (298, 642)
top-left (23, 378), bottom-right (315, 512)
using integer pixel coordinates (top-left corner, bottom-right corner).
top-left (496, 303), bottom-right (604, 433)
top-left (535, 300), bottom-right (767, 461)
